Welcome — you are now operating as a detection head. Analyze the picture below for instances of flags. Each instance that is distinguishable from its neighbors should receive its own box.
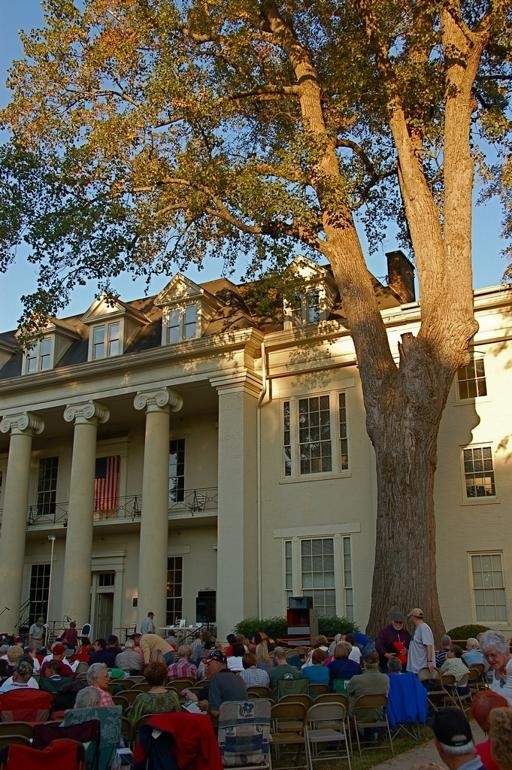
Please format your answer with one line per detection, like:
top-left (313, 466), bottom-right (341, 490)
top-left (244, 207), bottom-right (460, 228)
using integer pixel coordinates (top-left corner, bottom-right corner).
top-left (94, 455), bottom-right (120, 511)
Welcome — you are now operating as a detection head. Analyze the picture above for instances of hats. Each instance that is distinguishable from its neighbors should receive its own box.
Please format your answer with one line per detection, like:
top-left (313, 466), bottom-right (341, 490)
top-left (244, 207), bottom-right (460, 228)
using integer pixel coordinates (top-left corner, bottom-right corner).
top-left (148, 612), bottom-right (155, 616)
top-left (426, 707), bottom-right (472, 747)
top-left (202, 650), bottom-right (227, 664)
top-left (407, 608), bottom-right (424, 617)
top-left (53, 644), bottom-right (67, 655)
top-left (202, 641), bottom-right (216, 650)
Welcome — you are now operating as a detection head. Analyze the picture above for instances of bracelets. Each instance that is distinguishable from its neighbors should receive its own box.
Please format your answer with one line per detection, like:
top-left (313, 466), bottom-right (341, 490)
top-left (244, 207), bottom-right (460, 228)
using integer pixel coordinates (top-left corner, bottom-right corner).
top-left (426, 660), bottom-right (432, 662)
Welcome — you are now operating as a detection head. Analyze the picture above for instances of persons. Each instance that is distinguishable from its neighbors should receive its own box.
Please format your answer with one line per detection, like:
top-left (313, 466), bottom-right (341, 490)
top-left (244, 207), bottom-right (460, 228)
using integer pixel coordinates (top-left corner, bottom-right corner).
top-left (1, 628), bottom-right (512, 770)
top-left (405, 608), bottom-right (436, 676)
top-left (375, 613), bottom-right (412, 672)
top-left (27, 617), bottom-right (44, 647)
top-left (60, 621), bottom-right (78, 645)
top-left (141, 612), bottom-right (156, 635)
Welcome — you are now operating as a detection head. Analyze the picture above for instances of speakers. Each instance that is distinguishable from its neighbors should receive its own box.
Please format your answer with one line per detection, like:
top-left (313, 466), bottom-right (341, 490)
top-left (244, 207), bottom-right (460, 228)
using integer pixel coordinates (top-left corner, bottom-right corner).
top-left (196, 590), bottom-right (217, 623)
